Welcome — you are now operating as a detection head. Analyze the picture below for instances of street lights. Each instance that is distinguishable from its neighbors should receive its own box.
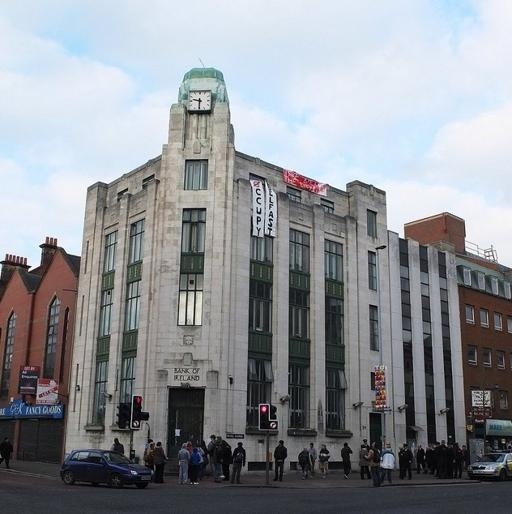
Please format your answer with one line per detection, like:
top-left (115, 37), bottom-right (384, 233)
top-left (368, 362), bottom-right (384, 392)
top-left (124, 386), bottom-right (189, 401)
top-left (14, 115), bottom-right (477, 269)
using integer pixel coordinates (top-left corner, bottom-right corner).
top-left (375, 243), bottom-right (387, 454)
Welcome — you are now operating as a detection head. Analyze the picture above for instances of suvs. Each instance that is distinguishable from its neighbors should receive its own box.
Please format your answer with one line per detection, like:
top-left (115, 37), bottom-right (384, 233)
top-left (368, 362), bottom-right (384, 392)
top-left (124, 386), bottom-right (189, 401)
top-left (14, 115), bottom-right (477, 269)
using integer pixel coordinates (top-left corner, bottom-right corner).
top-left (61, 449), bottom-right (153, 488)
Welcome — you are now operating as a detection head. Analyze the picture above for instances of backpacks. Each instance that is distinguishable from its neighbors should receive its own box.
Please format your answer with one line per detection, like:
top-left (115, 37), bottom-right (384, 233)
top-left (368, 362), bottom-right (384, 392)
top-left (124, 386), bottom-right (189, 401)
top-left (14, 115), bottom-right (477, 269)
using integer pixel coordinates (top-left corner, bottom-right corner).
top-left (235, 447), bottom-right (245, 465)
top-left (371, 448), bottom-right (380, 463)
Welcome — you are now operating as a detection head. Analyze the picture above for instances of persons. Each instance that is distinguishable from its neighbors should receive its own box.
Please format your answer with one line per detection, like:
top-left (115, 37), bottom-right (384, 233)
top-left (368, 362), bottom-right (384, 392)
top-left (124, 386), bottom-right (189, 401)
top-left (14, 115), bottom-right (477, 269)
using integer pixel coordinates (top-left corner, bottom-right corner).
top-left (0, 436), bottom-right (13, 469)
top-left (143, 433), bottom-right (247, 484)
top-left (299, 438), bottom-right (468, 488)
top-left (111, 436), bottom-right (125, 456)
top-left (273, 439), bottom-right (287, 481)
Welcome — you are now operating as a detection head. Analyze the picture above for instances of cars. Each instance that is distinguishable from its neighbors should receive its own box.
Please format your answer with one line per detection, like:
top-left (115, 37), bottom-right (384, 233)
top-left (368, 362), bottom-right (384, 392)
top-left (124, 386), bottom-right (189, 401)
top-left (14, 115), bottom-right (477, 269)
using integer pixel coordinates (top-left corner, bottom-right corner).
top-left (467, 451), bottom-right (511, 479)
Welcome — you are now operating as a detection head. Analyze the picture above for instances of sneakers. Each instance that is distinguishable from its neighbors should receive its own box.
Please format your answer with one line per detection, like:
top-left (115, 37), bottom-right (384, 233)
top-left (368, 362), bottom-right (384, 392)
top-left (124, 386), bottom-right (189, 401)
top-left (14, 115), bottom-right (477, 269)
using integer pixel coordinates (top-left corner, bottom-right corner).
top-left (273, 478), bottom-right (278, 481)
top-left (186, 482), bottom-right (193, 485)
top-left (344, 475), bottom-right (349, 480)
top-left (214, 480), bottom-right (221, 483)
top-left (222, 478), bottom-right (229, 481)
top-left (194, 482), bottom-right (200, 485)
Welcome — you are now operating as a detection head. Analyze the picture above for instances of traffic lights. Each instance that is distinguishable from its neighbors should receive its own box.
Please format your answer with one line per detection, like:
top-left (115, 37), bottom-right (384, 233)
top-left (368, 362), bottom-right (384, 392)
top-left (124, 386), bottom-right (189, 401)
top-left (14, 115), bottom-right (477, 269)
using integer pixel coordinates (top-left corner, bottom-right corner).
top-left (260, 406), bottom-right (269, 428)
top-left (114, 403), bottom-right (128, 427)
top-left (132, 395), bottom-right (149, 426)
